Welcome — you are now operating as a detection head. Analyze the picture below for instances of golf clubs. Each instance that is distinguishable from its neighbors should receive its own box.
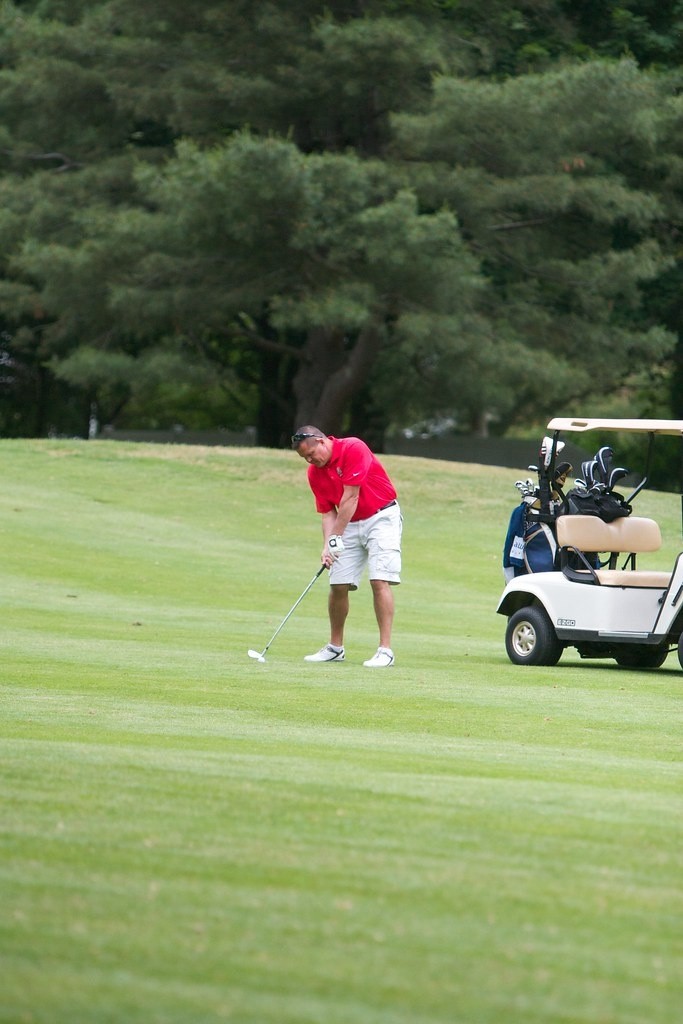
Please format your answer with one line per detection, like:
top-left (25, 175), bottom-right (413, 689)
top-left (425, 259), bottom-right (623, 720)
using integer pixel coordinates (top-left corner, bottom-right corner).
top-left (514, 465), bottom-right (538, 497)
top-left (247, 562), bottom-right (326, 660)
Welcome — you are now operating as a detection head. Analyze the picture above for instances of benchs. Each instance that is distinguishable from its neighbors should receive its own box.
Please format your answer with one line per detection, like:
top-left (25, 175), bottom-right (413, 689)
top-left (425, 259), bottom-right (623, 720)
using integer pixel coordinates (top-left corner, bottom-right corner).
top-left (556, 515), bottom-right (674, 587)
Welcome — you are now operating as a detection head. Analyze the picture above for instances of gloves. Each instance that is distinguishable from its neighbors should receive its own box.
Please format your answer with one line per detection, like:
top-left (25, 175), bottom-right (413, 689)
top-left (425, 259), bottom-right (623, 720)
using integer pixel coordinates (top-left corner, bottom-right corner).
top-left (328, 535), bottom-right (345, 560)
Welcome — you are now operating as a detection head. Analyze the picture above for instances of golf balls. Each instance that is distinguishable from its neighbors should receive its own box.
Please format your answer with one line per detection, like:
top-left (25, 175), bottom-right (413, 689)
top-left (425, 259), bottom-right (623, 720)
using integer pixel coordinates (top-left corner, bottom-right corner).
top-left (256, 657), bottom-right (265, 664)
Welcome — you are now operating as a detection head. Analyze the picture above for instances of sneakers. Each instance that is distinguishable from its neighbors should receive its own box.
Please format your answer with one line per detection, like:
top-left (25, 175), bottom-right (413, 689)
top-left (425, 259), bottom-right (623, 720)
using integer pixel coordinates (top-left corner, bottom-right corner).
top-left (363, 647), bottom-right (394, 667)
top-left (304, 643), bottom-right (345, 661)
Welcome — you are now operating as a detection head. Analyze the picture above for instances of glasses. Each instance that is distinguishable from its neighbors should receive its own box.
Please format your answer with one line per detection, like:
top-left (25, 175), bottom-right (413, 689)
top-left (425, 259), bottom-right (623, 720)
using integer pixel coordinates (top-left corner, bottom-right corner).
top-left (291, 433), bottom-right (323, 444)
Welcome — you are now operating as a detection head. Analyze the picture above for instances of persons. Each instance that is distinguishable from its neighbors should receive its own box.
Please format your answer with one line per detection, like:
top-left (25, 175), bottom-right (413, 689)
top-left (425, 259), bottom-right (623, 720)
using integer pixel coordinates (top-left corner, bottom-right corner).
top-left (291, 426), bottom-right (402, 667)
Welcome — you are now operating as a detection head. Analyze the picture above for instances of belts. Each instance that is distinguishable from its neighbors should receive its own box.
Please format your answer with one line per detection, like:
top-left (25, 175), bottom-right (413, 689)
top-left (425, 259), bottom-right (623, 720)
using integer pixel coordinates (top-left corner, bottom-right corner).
top-left (374, 501), bottom-right (396, 514)
